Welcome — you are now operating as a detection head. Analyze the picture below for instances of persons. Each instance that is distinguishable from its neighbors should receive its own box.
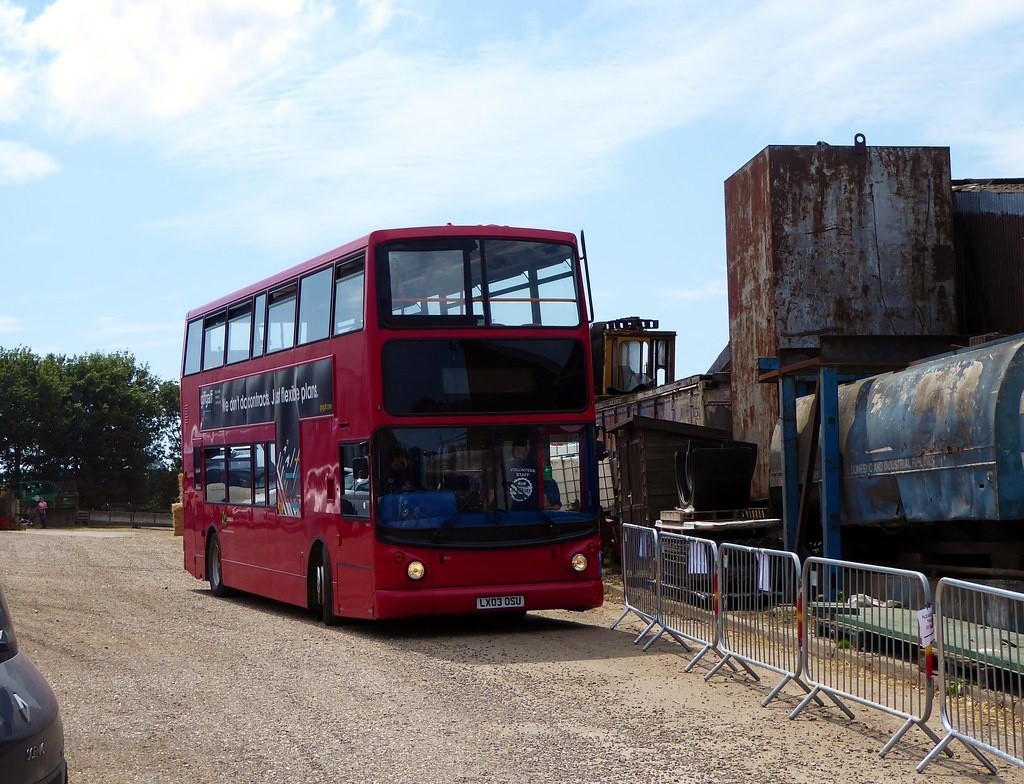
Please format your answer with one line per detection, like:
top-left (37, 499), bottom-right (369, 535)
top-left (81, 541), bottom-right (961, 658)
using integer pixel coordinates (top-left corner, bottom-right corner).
top-left (485, 439), bottom-right (562, 511)
top-left (353, 462), bottom-right (367, 480)
top-left (38, 496), bottom-right (47, 528)
top-left (391, 444), bottom-right (407, 473)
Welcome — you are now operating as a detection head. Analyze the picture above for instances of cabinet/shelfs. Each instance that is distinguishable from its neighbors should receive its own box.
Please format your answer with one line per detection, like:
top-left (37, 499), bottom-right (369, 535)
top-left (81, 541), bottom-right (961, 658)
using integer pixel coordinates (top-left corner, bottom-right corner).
top-left (655, 518), bottom-right (784, 611)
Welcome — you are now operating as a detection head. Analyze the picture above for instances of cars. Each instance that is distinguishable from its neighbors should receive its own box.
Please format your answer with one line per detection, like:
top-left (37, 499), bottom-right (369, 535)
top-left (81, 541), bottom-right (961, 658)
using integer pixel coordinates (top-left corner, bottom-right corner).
top-left (0, 582), bottom-right (68, 784)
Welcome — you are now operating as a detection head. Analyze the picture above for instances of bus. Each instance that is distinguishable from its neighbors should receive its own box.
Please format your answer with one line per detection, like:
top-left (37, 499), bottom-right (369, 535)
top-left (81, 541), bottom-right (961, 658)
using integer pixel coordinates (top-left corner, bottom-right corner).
top-left (179, 222), bottom-right (604, 638)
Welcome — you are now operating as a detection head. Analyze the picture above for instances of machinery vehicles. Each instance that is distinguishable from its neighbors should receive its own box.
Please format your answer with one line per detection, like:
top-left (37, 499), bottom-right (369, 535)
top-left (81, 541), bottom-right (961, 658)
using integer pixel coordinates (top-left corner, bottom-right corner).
top-left (589, 317), bottom-right (783, 580)
